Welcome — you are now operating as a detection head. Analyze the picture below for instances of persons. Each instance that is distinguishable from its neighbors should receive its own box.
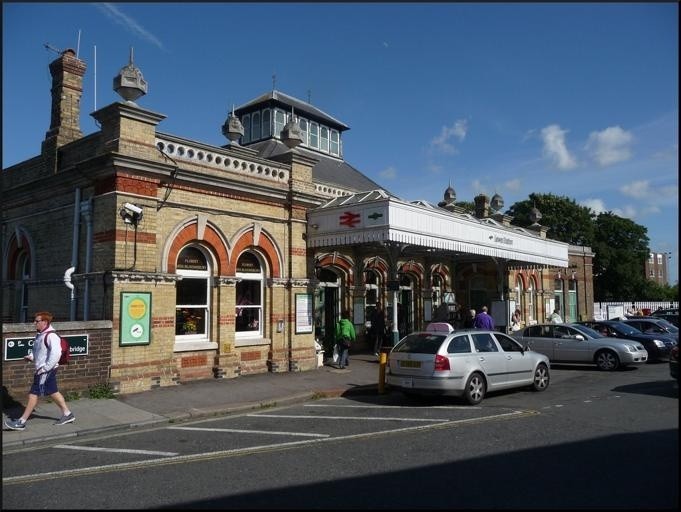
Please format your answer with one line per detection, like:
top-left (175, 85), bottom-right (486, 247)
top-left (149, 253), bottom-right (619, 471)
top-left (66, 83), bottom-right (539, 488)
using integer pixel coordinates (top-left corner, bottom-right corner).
top-left (547, 309), bottom-right (563, 324)
top-left (473, 304), bottom-right (495, 331)
top-left (398, 303), bottom-right (407, 332)
top-left (4, 310), bottom-right (77, 430)
top-left (334, 310), bottom-right (354, 368)
top-left (365, 301), bottom-right (387, 356)
top-left (509, 309), bottom-right (525, 332)
top-left (465, 309), bottom-right (476, 328)
top-left (637, 309), bottom-right (644, 316)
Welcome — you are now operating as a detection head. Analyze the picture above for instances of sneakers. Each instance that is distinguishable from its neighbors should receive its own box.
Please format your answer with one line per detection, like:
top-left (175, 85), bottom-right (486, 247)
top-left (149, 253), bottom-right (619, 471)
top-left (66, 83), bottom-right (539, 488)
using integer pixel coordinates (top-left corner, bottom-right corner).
top-left (55, 412), bottom-right (76, 425)
top-left (5, 419), bottom-right (26, 431)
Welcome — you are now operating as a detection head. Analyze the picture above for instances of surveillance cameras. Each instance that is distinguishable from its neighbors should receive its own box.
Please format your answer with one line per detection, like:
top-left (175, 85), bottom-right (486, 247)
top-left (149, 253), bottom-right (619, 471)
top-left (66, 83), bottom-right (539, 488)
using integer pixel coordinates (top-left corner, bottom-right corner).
top-left (124, 202), bottom-right (143, 215)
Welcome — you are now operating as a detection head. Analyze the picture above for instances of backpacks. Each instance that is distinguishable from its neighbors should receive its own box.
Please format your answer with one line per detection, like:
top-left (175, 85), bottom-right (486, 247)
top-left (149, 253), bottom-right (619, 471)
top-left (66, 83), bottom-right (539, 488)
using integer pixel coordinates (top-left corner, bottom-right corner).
top-left (45, 331), bottom-right (69, 365)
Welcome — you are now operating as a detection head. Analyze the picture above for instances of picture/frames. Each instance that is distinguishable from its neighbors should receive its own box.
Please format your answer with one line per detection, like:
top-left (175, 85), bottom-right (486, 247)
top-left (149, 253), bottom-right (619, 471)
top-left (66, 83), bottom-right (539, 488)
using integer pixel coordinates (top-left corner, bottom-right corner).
top-left (353, 296), bottom-right (366, 326)
top-left (119, 290), bottom-right (153, 347)
top-left (294, 292), bottom-right (314, 334)
top-left (423, 297), bottom-right (432, 323)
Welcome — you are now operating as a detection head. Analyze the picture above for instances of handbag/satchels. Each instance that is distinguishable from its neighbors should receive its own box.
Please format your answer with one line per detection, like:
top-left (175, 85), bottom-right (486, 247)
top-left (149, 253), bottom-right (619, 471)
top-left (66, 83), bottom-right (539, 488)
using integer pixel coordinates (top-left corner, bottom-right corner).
top-left (342, 336), bottom-right (352, 348)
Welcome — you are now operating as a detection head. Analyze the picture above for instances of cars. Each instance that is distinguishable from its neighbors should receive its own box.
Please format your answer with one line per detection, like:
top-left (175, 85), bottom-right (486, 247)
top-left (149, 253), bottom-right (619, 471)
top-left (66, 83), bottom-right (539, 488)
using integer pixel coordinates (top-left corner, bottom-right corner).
top-left (667, 343), bottom-right (680, 380)
top-left (610, 309), bottom-right (679, 342)
top-left (509, 325), bottom-right (649, 372)
top-left (581, 321), bottom-right (676, 361)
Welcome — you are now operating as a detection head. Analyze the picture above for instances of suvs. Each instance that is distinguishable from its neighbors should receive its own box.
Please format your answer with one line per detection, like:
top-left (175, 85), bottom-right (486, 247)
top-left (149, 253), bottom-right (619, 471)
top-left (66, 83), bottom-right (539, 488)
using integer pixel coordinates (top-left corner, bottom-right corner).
top-left (384, 320), bottom-right (552, 404)
